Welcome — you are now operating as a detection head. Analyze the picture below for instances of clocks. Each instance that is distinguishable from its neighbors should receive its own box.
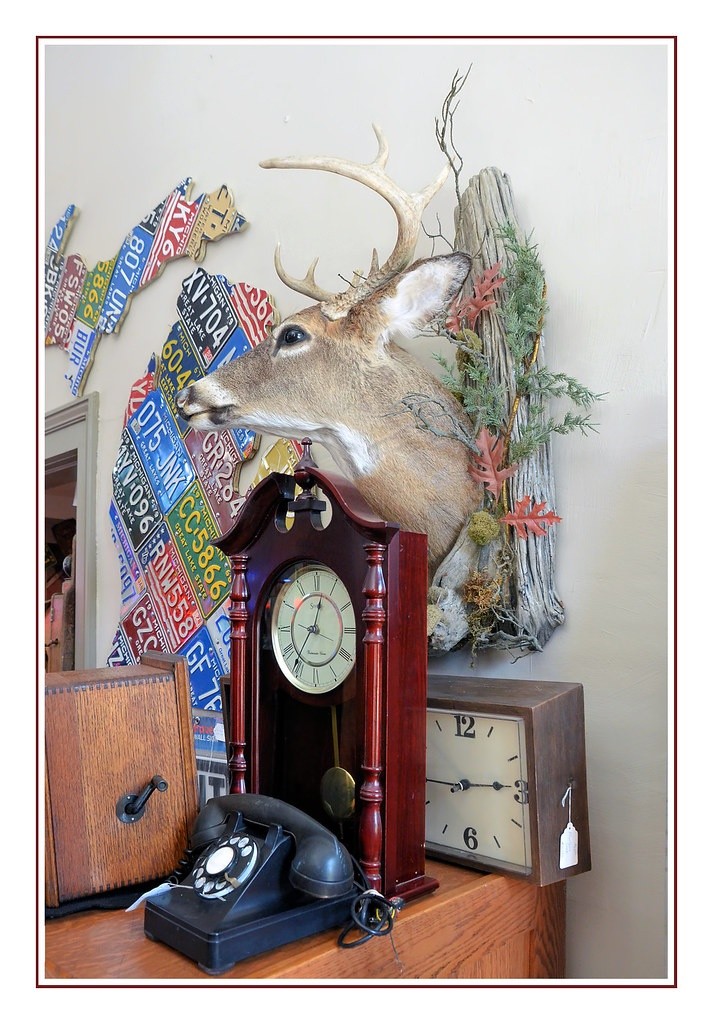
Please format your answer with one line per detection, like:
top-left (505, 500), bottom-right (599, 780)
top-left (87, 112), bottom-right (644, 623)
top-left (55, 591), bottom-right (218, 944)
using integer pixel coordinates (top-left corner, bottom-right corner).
top-left (425, 676), bottom-right (593, 887)
top-left (210, 438), bottom-right (441, 903)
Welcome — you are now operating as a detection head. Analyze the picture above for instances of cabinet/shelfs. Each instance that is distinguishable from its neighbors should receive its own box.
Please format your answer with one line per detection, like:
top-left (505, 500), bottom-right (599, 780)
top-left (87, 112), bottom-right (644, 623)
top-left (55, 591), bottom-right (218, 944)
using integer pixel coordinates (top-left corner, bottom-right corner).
top-left (45, 858), bottom-right (566, 979)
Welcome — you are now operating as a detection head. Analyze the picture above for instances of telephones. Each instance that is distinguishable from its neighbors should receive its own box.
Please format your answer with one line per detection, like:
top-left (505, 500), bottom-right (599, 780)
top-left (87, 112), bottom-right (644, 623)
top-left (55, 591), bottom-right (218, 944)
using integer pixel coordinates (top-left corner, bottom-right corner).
top-left (142, 792), bottom-right (368, 973)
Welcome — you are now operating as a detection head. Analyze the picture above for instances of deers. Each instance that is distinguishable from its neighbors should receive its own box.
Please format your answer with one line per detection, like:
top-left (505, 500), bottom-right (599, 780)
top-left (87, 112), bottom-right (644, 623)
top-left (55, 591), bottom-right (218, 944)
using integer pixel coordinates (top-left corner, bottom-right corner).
top-left (173, 122), bottom-right (479, 582)
